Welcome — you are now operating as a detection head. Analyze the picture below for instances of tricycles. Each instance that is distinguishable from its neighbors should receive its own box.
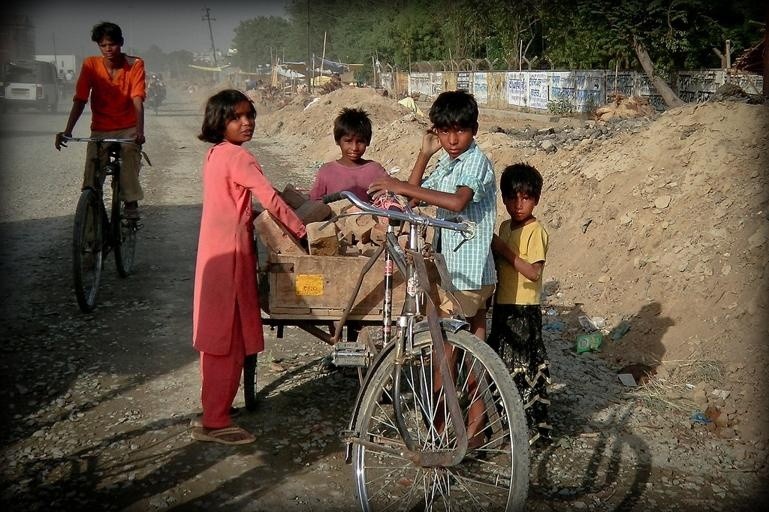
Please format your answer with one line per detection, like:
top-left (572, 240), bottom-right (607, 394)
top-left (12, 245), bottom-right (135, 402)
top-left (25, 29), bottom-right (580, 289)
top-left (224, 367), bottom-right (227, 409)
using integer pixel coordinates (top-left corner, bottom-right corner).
top-left (239, 189), bottom-right (532, 512)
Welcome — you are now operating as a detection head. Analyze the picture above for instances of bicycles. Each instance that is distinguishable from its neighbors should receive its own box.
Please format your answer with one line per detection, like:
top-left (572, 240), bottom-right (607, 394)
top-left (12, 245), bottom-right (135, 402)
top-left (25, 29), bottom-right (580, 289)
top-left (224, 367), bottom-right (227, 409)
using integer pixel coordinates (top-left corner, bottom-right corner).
top-left (55, 131), bottom-right (152, 312)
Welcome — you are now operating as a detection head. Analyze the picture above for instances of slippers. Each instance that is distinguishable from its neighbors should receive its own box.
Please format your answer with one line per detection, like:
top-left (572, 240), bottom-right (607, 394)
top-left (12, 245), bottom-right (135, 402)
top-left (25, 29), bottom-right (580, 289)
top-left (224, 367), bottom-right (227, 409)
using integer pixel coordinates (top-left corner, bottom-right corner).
top-left (189, 407), bottom-right (240, 427)
top-left (191, 425), bottom-right (257, 445)
top-left (123, 207), bottom-right (141, 219)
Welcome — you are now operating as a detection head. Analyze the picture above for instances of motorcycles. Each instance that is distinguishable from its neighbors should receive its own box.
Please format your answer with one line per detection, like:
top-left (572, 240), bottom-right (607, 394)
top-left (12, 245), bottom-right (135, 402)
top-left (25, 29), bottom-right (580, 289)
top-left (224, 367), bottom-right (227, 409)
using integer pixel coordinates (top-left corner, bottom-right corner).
top-left (144, 82), bottom-right (168, 114)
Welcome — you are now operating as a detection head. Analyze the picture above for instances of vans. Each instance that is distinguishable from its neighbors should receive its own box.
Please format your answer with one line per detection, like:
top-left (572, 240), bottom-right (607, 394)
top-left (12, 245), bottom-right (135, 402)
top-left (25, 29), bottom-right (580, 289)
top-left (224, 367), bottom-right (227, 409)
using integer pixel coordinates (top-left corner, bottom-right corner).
top-left (4, 59), bottom-right (59, 113)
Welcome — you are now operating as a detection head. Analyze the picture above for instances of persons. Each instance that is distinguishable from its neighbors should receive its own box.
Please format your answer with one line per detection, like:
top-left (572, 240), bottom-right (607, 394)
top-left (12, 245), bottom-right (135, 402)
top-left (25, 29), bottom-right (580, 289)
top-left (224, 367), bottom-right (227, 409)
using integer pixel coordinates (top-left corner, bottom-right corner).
top-left (52, 21), bottom-right (146, 273)
top-left (190, 88), bottom-right (309, 444)
top-left (65, 70), bottom-right (75, 81)
top-left (56, 69), bottom-right (65, 81)
top-left (481, 160), bottom-right (552, 449)
top-left (365, 87), bottom-right (501, 452)
top-left (308, 105), bottom-right (393, 371)
top-left (146, 74), bottom-right (164, 90)
